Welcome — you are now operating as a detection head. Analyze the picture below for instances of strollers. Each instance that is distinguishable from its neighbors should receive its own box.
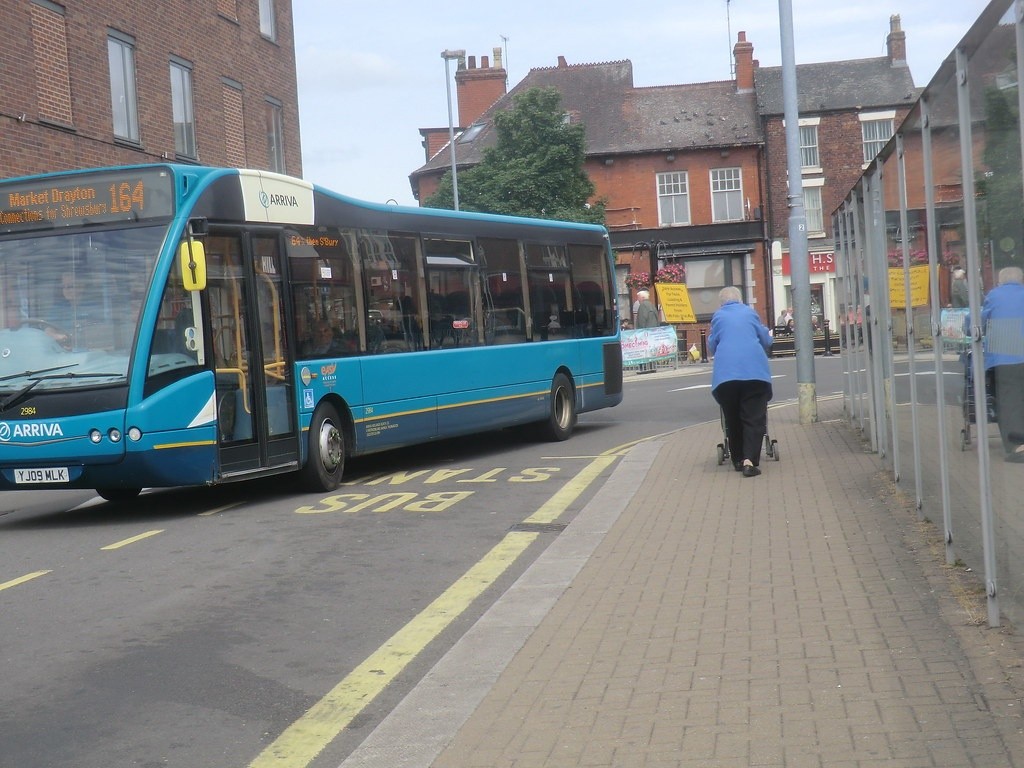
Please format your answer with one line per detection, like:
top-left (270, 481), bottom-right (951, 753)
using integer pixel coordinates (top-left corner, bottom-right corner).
top-left (716, 401), bottom-right (780, 466)
top-left (958, 349), bottom-right (997, 451)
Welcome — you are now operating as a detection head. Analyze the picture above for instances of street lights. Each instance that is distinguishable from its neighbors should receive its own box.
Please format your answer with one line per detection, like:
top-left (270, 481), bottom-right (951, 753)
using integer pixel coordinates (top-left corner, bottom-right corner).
top-left (439, 48), bottom-right (469, 209)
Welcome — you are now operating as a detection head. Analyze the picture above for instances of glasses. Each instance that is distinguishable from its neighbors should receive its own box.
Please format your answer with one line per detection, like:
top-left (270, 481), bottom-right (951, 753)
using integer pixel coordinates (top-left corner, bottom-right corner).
top-left (61, 283), bottom-right (75, 289)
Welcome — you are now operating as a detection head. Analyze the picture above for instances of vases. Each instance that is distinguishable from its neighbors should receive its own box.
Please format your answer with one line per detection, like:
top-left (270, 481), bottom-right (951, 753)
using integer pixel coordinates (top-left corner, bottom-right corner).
top-left (635, 284), bottom-right (650, 290)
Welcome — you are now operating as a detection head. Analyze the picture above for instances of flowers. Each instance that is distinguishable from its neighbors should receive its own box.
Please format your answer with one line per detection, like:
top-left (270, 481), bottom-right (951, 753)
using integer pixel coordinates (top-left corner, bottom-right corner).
top-left (625, 272), bottom-right (650, 286)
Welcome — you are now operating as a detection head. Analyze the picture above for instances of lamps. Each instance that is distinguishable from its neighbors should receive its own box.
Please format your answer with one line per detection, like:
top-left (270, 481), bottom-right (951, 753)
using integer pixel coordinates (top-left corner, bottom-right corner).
top-left (720, 149), bottom-right (730, 157)
top-left (666, 152), bottom-right (675, 162)
top-left (160, 151), bottom-right (168, 158)
top-left (602, 156), bottom-right (614, 167)
top-left (18, 113), bottom-right (26, 123)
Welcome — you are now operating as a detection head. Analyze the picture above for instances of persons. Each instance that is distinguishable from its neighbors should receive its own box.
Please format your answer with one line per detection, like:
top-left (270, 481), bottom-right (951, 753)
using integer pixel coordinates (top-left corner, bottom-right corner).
top-left (297, 308), bottom-right (310, 356)
top-left (838, 304), bottom-right (848, 349)
top-left (848, 305), bottom-right (855, 346)
top-left (961, 266), bottom-right (1024, 463)
top-left (636, 291), bottom-right (659, 370)
top-left (706, 284), bottom-right (774, 477)
top-left (620, 319), bottom-right (631, 330)
top-left (785, 319), bottom-right (795, 336)
top-left (157, 307), bottom-right (229, 369)
top-left (811, 315), bottom-right (823, 333)
top-left (305, 320), bottom-right (353, 358)
top-left (777, 310), bottom-right (788, 337)
top-left (430, 292), bottom-right (459, 345)
top-left (784, 307), bottom-right (794, 326)
top-left (856, 305), bottom-right (863, 345)
top-left (43, 262), bottom-right (100, 353)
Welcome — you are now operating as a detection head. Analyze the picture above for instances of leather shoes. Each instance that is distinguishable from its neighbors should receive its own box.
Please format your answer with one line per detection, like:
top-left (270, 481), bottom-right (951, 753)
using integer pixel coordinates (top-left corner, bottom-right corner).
top-left (1003, 449), bottom-right (1024, 463)
top-left (735, 461), bottom-right (744, 471)
top-left (743, 464), bottom-right (764, 476)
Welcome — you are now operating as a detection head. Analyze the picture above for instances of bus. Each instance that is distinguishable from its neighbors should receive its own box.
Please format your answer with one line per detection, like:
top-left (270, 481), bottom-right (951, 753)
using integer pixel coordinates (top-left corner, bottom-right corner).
top-left (0, 163), bottom-right (628, 501)
